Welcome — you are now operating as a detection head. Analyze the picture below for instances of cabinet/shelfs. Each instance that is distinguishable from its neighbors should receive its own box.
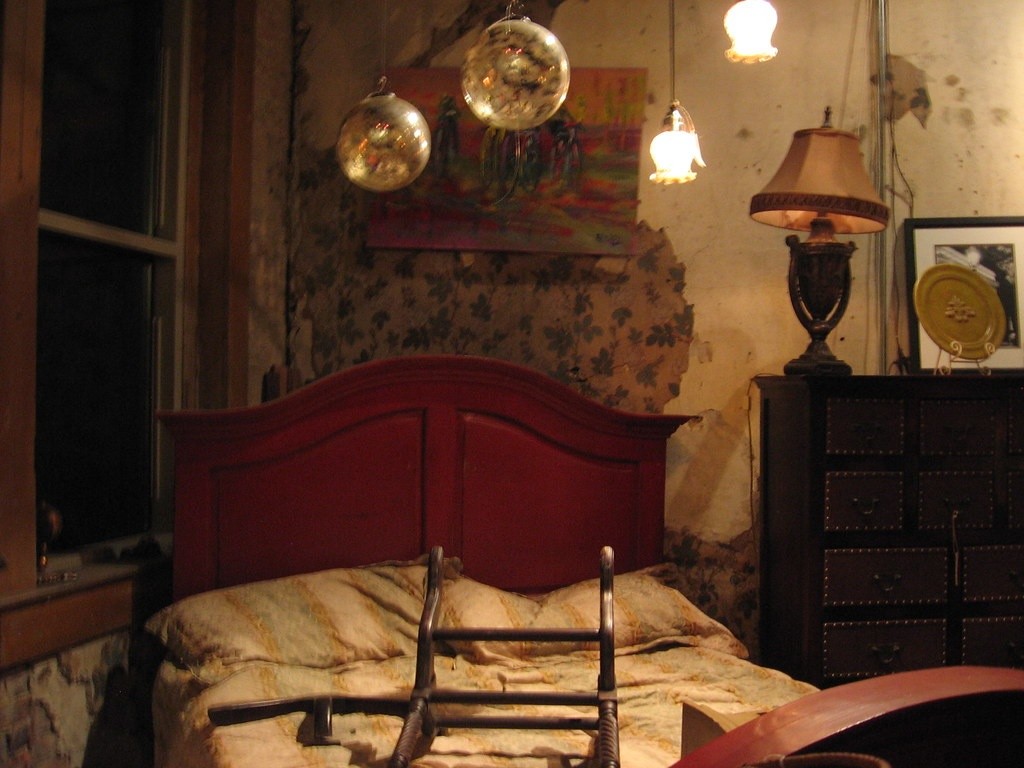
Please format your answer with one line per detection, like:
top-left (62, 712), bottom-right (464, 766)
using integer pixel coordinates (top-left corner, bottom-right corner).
top-left (752, 378), bottom-right (1024, 767)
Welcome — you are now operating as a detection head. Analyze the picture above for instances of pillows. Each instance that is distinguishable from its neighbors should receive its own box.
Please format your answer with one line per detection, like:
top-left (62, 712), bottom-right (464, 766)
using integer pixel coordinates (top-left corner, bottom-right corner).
top-left (146, 554), bottom-right (425, 686)
top-left (436, 557), bottom-right (748, 659)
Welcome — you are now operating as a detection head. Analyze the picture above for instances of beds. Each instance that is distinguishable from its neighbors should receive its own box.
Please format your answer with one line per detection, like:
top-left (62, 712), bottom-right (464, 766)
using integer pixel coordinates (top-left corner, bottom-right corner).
top-left (158, 353), bottom-right (1024, 768)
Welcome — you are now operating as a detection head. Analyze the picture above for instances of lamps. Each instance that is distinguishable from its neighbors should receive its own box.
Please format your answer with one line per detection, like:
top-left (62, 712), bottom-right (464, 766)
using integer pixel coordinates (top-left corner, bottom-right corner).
top-left (723, 0), bottom-right (778, 65)
top-left (460, 0), bottom-right (571, 132)
top-left (335, 0), bottom-right (431, 193)
top-left (647, 0), bottom-right (708, 187)
top-left (748, 106), bottom-right (889, 377)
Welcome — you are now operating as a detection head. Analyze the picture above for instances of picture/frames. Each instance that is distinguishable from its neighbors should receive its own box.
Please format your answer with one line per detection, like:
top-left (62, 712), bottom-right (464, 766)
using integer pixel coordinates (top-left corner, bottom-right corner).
top-left (905, 215), bottom-right (1024, 376)
